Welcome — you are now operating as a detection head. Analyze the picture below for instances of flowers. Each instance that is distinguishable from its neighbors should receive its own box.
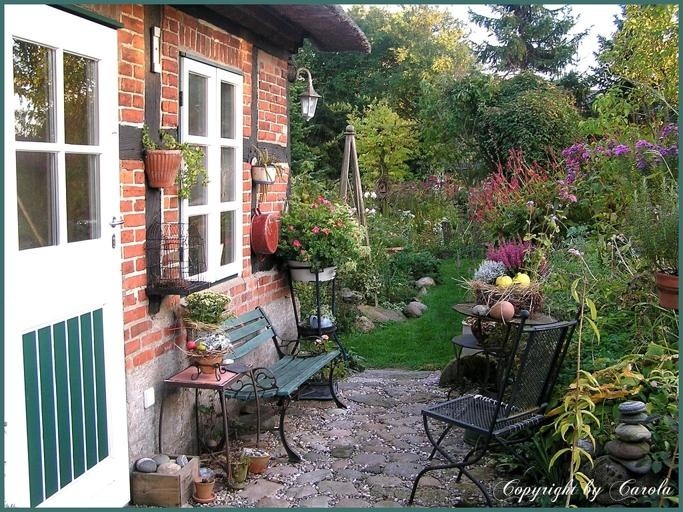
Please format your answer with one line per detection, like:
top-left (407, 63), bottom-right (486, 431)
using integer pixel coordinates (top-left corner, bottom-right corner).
top-left (185, 288), bottom-right (231, 323)
top-left (304, 334), bottom-right (347, 383)
top-left (268, 194), bottom-right (361, 274)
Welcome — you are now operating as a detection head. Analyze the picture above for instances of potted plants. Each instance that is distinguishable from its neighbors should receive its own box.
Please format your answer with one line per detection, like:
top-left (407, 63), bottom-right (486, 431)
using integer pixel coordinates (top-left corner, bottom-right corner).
top-left (192, 477), bottom-right (218, 503)
top-left (250, 144), bottom-right (285, 203)
top-left (230, 453), bottom-right (253, 483)
top-left (624, 179), bottom-right (679, 310)
top-left (141, 122), bottom-right (209, 200)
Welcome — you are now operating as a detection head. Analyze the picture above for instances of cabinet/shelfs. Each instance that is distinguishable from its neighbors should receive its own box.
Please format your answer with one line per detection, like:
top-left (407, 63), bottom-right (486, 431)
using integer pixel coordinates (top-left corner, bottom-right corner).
top-left (284, 264), bottom-right (349, 369)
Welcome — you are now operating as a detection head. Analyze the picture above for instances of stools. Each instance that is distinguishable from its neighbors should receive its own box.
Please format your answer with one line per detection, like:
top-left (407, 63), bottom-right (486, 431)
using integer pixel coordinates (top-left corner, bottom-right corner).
top-left (447, 334), bottom-right (512, 401)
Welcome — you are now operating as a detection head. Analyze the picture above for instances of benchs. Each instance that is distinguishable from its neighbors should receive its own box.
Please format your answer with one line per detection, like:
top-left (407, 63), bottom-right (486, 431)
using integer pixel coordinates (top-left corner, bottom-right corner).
top-left (188, 305), bottom-right (347, 462)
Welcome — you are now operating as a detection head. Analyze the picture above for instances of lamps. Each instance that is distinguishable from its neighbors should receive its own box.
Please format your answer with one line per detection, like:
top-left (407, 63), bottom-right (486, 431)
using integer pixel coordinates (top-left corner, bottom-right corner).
top-left (287, 67), bottom-right (322, 122)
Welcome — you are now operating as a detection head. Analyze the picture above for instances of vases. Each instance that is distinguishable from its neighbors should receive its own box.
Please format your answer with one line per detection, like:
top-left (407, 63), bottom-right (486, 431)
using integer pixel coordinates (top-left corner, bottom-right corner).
top-left (298, 378), bottom-right (339, 401)
top-left (288, 259), bottom-right (337, 281)
top-left (249, 455), bottom-right (271, 474)
top-left (184, 325), bottom-right (215, 366)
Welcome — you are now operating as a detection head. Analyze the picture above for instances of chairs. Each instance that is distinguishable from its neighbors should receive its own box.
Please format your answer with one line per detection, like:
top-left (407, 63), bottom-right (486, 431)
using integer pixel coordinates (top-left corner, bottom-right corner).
top-left (408, 303), bottom-right (587, 506)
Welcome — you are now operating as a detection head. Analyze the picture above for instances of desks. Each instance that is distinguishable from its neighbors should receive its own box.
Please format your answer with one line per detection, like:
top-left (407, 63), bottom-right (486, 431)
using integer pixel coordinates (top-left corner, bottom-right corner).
top-left (158, 363), bottom-right (261, 479)
top-left (451, 302), bottom-right (560, 447)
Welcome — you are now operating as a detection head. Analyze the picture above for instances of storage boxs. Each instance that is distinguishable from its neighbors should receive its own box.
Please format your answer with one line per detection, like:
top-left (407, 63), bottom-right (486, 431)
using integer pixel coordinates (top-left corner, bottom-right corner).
top-left (131, 454), bottom-right (200, 508)
